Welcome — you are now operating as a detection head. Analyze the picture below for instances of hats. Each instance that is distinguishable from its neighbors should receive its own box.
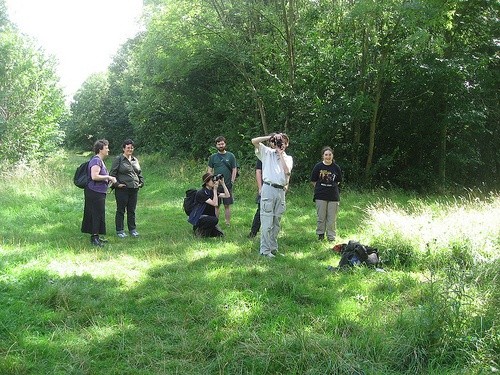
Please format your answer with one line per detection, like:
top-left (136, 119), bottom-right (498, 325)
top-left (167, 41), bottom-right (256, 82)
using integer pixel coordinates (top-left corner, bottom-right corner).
top-left (202, 173), bottom-right (214, 187)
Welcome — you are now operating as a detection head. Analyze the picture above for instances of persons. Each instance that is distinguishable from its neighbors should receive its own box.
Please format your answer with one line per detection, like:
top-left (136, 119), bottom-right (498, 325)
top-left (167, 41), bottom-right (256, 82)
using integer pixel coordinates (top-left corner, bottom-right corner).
top-left (310, 146), bottom-right (343, 244)
top-left (206, 136), bottom-right (237, 227)
top-left (108, 139), bottom-right (144, 238)
top-left (188, 174), bottom-right (231, 239)
top-left (80, 139), bottom-right (117, 248)
top-left (247, 133), bottom-right (293, 259)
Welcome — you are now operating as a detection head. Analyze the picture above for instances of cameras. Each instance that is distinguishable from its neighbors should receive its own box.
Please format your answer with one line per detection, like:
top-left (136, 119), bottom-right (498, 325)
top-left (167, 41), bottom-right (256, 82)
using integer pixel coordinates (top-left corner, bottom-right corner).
top-left (213, 174), bottom-right (224, 181)
top-left (277, 140), bottom-right (283, 150)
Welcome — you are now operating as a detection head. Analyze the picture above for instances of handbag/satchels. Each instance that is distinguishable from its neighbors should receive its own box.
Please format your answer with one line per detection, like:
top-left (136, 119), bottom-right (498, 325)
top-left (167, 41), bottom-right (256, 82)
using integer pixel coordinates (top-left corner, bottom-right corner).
top-left (231, 167), bottom-right (240, 178)
top-left (108, 172), bottom-right (115, 189)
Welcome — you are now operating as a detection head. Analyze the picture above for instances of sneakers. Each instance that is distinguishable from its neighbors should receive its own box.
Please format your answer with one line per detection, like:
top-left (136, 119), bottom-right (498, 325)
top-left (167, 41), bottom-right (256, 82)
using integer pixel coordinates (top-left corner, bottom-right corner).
top-left (261, 253), bottom-right (275, 258)
top-left (130, 231), bottom-right (139, 237)
top-left (116, 232), bottom-right (128, 237)
top-left (195, 227), bottom-right (203, 237)
top-left (272, 249), bottom-right (285, 257)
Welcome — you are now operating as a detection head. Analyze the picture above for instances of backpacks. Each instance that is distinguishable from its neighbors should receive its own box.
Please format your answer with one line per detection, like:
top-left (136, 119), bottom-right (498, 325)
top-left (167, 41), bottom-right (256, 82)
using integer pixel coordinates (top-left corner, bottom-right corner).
top-left (74, 157), bottom-right (103, 188)
top-left (338, 240), bottom-right (379, 271)
top-left (183, 189), bottom-right (210, 216)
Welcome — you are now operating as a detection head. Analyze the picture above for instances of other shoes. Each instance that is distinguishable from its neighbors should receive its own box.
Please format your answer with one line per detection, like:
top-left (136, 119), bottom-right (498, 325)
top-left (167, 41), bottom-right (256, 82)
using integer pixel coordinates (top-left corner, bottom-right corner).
top-left (91, 235), bottom-right (101, 247)
top-left (318, 234), bottom-right (324, 241)
top-left (247, 229), bottom-right (257, 239)
top-left (98, 237), bottom-right (108, 243)
top-left (226, 223), bottom-right (230, 228)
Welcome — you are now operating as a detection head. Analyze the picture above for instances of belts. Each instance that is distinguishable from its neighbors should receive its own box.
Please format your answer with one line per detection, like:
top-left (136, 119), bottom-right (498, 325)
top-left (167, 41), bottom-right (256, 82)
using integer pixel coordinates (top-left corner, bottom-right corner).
top-left (262, 181), bottom-right (284, 189)
